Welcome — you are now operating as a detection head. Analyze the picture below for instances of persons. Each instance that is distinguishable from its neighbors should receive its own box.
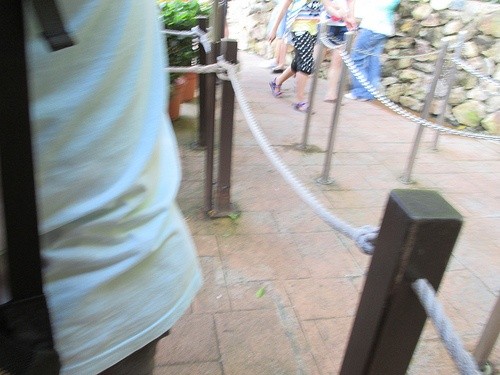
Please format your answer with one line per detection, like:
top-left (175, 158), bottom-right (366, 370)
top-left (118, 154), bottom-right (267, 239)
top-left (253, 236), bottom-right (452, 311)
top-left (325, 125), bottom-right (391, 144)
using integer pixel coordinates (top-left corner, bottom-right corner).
top-left (267, 0), bottom-right (403, 111)
top-left (0, 0), bottom-right (206, 375)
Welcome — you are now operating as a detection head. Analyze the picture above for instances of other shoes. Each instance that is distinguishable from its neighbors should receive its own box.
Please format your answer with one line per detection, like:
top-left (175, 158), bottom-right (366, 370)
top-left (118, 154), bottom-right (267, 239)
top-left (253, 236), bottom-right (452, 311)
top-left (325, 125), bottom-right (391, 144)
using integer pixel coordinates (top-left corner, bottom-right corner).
top-left (271, 64), bottom-right (284, 74)
top-left (341, 93), bottom-right (370, 100)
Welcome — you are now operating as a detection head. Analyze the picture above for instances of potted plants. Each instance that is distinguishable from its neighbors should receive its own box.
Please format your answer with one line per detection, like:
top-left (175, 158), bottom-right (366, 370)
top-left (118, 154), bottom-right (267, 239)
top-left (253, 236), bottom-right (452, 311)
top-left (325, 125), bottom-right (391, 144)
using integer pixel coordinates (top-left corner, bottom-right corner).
top-left (161, 0), bottom-right (209, 121)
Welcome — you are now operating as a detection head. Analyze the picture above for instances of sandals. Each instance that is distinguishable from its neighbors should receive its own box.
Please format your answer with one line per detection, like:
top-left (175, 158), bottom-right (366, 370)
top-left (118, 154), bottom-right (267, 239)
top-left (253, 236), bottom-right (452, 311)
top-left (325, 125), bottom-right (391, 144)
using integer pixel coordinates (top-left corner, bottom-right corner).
top-left (268, 78), bottom-right (285, 99)
top-left (290, 101), bottom-right (316, 115)
